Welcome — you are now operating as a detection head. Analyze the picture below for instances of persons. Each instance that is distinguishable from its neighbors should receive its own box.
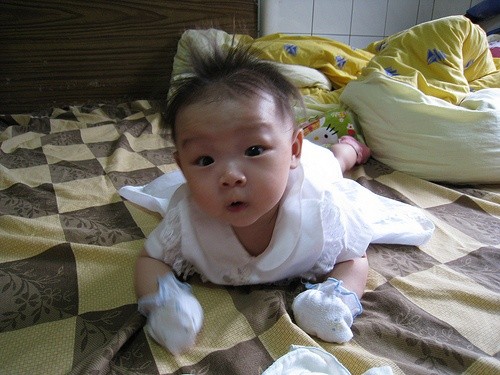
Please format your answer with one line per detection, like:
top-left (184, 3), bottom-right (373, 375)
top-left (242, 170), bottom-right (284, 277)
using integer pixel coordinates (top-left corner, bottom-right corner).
top-left (117, 45), bottom-right (434, 357)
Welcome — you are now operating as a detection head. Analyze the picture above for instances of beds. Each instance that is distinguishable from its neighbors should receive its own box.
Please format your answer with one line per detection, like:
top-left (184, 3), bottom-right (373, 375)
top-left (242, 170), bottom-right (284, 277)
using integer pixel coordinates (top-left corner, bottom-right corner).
top-left (0, 100), bottom-right (500, 375)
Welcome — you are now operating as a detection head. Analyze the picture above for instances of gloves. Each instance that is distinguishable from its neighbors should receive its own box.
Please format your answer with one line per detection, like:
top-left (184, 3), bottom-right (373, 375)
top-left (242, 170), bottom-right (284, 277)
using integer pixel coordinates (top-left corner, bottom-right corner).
top-left (292, 277), bottom-right (363, 344)
top-left (137, 273), bottom-right (204, 356)
